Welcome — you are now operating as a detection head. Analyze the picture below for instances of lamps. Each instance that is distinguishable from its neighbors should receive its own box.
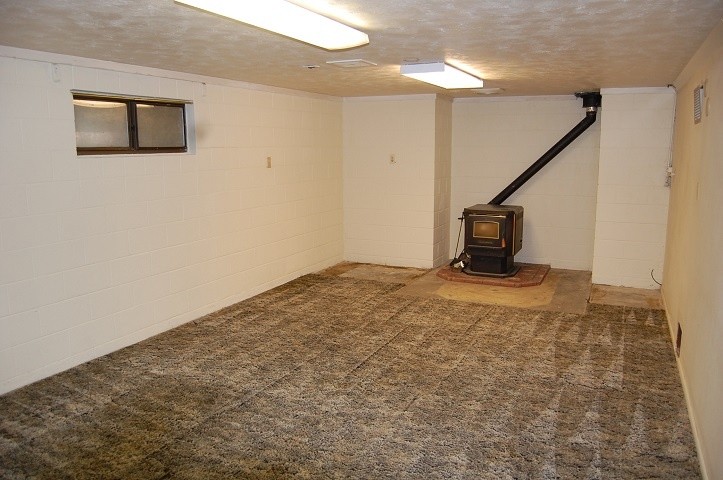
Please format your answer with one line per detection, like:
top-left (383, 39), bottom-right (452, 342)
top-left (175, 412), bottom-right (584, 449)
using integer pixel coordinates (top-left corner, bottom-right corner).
top-left (174, 0), bottom-right (369, 51)
top-left (400, 63), bottom-right (483, 90)
